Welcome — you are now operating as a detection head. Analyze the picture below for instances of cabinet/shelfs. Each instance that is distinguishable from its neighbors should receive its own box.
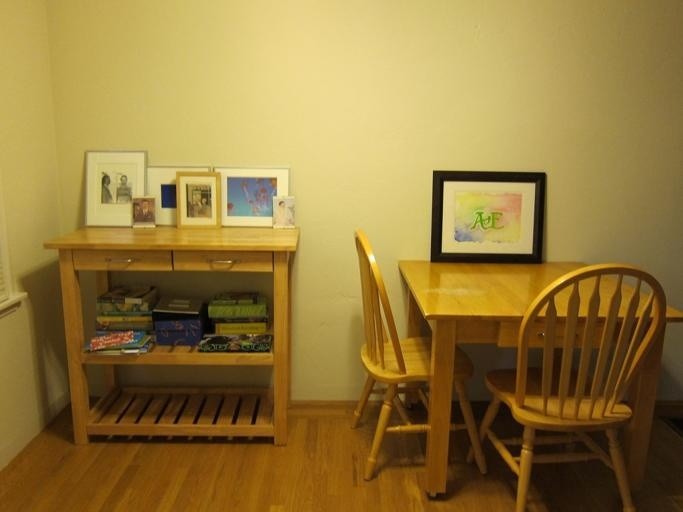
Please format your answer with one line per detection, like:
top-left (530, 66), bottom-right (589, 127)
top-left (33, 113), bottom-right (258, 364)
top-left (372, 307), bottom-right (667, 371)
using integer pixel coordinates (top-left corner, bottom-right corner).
top-left (42, 227), bottom-right (300, 446)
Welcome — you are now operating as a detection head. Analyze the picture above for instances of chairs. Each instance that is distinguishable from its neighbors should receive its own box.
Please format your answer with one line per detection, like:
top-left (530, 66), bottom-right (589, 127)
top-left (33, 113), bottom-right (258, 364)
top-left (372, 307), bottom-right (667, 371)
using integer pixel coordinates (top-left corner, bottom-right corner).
top-left (350, 227), bottom-right (488, 481)
top-left (466, 262), bottom-right (666, 512)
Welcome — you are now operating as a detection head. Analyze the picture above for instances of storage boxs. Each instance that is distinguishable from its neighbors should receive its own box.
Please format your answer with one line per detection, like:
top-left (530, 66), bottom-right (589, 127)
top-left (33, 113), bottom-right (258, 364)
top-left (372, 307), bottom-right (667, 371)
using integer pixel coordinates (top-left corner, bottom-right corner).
top-left (154, 301), bottom-right (209, 346)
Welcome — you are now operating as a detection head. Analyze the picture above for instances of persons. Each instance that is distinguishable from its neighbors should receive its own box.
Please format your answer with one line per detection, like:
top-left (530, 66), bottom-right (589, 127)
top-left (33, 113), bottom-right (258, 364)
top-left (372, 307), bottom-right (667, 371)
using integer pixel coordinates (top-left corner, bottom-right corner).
top-left (100, 175), bottom-right (113, 203)
top-left (134, 200), bottom-right (153, 219)
top-left (132, 202), bottom-right (142, 221)
top-left (273, 200), bottom-right (292, 226)
top-left (115, 175), bottom-right (133, 204)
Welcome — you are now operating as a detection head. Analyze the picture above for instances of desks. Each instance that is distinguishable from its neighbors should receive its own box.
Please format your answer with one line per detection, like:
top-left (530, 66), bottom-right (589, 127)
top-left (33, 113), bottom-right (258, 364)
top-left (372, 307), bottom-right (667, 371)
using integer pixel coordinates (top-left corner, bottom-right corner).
top-left (398, 261), bottom-right (683, 499)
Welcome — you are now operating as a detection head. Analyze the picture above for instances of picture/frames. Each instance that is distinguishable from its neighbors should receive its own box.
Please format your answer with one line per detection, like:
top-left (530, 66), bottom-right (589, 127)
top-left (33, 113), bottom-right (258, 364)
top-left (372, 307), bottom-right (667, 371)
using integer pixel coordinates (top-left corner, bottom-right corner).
top-left (429, 170), bottom-right (546, 263)
top-left (175, 170), bottom-right (222, 232)
top-left (212, 166), bottom-right (291, 228)
top-left (146, 164), bottom-right (213, 227)
top-left (83, 149), bottom-right (147, 229)
